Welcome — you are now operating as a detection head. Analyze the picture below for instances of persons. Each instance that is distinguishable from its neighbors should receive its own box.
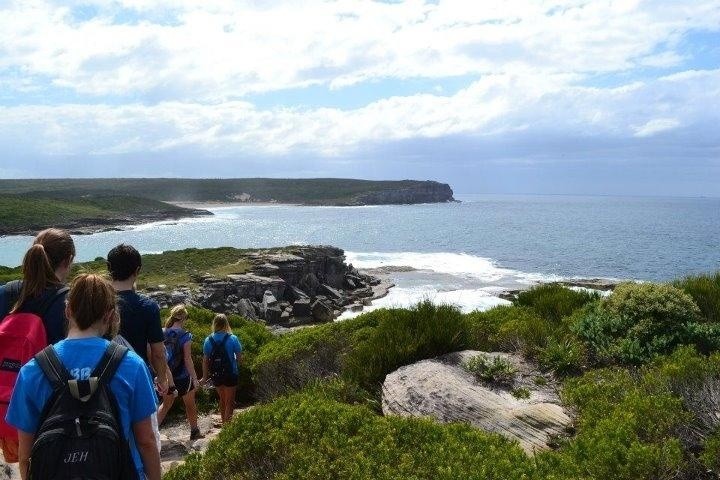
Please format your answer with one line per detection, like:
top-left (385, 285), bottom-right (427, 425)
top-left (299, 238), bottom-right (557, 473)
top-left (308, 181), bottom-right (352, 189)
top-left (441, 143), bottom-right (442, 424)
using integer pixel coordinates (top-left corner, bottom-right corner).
top-left (102, 244), bottom-right (169, 395)
top-left (148, 343), bottom-right (179, 398)
top-left (0, 227), bottom-right (75, 464)
top-left (4, 272), bottom-right (161, 480)
top-left (198, 313), bottom-right (243, 425)
top-left (157, 304), bottom-right (205, 441)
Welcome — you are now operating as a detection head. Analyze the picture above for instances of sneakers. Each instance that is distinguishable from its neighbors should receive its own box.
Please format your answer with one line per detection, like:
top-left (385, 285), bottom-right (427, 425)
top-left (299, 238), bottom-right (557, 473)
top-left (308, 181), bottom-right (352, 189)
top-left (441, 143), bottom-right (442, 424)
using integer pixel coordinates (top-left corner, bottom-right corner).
top-left (190, 433), bottom-right (205, 441)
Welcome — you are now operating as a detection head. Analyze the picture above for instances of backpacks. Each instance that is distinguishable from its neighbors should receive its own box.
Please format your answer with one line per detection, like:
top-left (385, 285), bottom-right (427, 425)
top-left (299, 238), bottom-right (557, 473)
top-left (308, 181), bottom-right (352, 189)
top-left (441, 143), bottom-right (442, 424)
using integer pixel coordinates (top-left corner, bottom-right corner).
top-left (162, 327), bottom-right (190, 377)
top-left (0, 280), bottom-right (71, 442)
top-left (25, 340), bottom-right (140, 480)
top-left (208, 331), bottom-right (232, 379)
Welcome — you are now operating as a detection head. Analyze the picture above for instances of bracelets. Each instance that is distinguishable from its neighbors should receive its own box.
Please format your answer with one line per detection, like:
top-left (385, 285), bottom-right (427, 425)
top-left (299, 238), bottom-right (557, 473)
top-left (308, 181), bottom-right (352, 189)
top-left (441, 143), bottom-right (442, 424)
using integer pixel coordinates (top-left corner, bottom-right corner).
top-left (167, 385), bottom-right (176, 394)
top-left (192, 376), bottom-right (197, 381)
